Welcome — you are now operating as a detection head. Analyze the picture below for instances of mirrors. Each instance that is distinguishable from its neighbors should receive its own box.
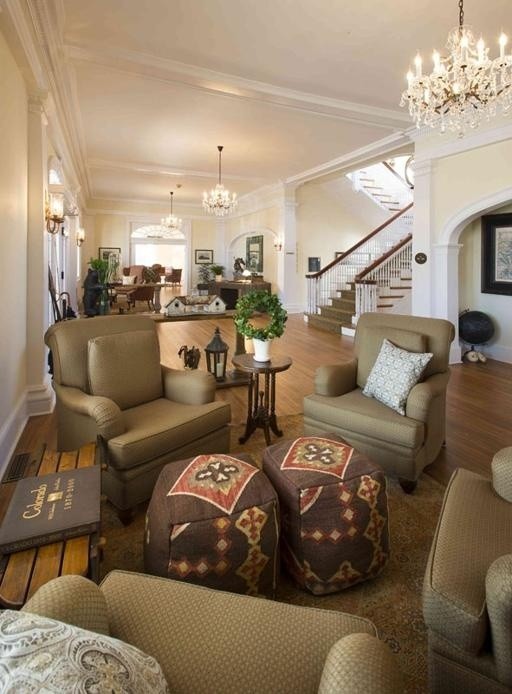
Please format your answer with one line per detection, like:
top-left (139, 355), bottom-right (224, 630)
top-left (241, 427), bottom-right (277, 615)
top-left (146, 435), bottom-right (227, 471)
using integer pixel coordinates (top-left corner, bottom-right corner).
top-left (245, 235), bottom-right (264, 271)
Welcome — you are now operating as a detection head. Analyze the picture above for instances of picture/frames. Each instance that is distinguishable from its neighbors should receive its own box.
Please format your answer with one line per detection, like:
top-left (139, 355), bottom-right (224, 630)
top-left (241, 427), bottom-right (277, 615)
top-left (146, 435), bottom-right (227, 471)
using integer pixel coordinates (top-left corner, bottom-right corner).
top-left (194, 249), bottom-right (214, 263)
top-left (99, 247), bottom-right (121, 263)
top-left (481, 211), bottom-right (512, 294)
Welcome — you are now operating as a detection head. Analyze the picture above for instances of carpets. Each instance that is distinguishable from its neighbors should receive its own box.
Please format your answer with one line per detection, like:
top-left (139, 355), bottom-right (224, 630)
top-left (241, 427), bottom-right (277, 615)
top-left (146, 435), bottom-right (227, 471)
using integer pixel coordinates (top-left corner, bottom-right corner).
top-left (93, 413), bottom-right (446, 694)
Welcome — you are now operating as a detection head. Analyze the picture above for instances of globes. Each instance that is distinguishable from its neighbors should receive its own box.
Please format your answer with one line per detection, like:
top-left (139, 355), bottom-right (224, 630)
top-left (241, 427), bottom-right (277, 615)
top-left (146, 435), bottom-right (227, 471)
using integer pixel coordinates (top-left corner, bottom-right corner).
top-left (458, 308), bottom-right (495, 365)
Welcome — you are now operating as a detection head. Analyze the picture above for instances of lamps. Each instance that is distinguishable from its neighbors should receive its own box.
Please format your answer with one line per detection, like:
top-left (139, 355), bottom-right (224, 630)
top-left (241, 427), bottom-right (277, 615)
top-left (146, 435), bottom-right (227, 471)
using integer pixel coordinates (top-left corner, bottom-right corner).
top-left (76, 226), bottom-right (86, 246)
top-left (398, 1), bottom-right (512, 138)
top-left (45, 189), bottom-right (66, 235)
top-left (160, 190), bottom-right (183, 234)
top-left (201, 144), bottom-right (239, 216)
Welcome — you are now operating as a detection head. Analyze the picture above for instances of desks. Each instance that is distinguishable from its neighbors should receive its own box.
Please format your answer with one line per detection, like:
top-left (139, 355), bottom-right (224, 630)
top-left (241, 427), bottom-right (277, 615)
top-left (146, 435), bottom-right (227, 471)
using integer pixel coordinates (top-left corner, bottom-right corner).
top-left (208, 282), bottom-right (271, 313)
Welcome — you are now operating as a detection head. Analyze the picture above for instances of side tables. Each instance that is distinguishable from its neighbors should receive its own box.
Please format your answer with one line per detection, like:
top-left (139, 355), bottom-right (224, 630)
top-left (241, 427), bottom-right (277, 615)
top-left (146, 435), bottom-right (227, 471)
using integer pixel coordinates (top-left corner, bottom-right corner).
top-left (232, 352), bottom-right (292, 446)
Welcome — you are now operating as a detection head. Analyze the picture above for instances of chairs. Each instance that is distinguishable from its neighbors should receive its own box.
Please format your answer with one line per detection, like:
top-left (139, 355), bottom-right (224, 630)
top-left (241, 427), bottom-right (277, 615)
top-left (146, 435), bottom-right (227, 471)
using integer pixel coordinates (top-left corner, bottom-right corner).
top-left (44, 313), bottom-right (232, 527)
top-left (1, 569), bottom-right (402, 694)
top-left (106, 263), bottom-right (182, 312)
top-left (423, 445), bottom-right (512, 694)
top-left (303, 311), bottom-right (453, 495)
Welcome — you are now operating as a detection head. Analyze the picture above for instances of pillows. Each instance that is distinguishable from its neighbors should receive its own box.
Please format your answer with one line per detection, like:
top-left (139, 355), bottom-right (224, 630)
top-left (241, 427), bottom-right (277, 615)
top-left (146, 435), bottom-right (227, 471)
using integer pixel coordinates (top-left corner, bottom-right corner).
top-left (1, 607), bottom-right (170, 694)
top-left (358, 337), bottom-right (434, 417)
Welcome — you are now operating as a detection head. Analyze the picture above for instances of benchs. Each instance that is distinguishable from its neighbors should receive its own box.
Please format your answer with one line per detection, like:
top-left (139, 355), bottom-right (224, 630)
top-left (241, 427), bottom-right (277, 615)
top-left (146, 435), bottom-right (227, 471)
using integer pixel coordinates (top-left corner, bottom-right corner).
top-left (0, 441), bottom-right (101, 607)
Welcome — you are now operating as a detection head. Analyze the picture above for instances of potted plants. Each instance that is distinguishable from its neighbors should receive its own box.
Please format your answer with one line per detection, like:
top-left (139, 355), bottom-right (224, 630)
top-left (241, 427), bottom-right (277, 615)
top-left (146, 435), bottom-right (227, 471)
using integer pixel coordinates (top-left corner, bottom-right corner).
top-left (236, 290), bottom-right (287, 362)
top-left (211, 265), bottom-right (225, 282)
